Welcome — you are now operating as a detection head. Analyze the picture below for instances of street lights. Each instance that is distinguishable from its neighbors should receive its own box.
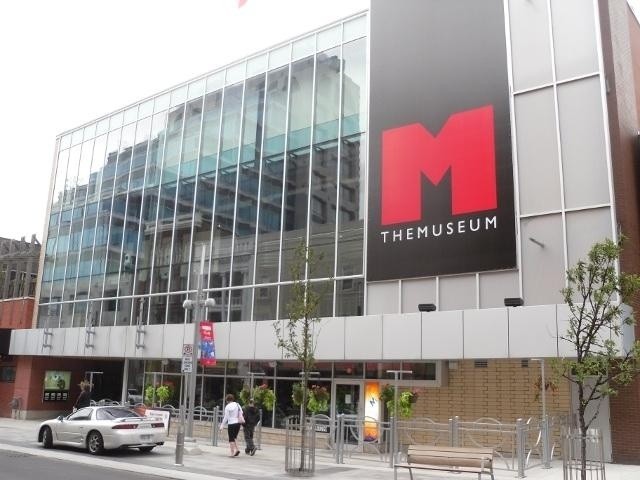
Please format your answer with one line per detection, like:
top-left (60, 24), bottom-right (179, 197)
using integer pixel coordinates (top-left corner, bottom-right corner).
top-left (181, 274), bottom-right (216, 441)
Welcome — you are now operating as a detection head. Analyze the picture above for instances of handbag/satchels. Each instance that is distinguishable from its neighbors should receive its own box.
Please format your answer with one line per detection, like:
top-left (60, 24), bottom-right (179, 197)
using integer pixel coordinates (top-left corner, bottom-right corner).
top-left (238, 411), bottom-right (244, 423)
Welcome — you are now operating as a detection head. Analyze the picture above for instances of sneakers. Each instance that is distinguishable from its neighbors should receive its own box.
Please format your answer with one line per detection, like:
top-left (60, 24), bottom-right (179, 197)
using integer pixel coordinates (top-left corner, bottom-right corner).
top-left (228, 447), bottom-right (258, 457)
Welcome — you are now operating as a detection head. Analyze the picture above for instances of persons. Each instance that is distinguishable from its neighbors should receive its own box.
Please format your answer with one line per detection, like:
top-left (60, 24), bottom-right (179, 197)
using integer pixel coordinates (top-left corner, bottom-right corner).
top-left (218, 393), bottom-right (245, 459)
top-left (71, 379), bottom-right (89, 411)
top-left (243, 396), bottom-right (261, 457)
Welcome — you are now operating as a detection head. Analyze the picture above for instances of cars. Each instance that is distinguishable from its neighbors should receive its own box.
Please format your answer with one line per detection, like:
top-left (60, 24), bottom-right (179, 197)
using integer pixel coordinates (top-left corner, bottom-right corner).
top-left (127, 389), bottom-right (143, 405)
top-left (36, 404), bottom-right (166, 454)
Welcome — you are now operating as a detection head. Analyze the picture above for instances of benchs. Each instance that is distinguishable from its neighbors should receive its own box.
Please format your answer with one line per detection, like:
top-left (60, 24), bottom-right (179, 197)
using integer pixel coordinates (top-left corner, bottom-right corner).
top-left (393, 444), bottom-right (495, 480)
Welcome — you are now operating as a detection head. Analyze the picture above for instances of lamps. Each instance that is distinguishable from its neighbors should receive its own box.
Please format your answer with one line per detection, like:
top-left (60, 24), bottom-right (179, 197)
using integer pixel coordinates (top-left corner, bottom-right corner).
top-left (504, 297), bottom-right (523, 307)
top-left (418, 303), bottom-right (435, 312)
